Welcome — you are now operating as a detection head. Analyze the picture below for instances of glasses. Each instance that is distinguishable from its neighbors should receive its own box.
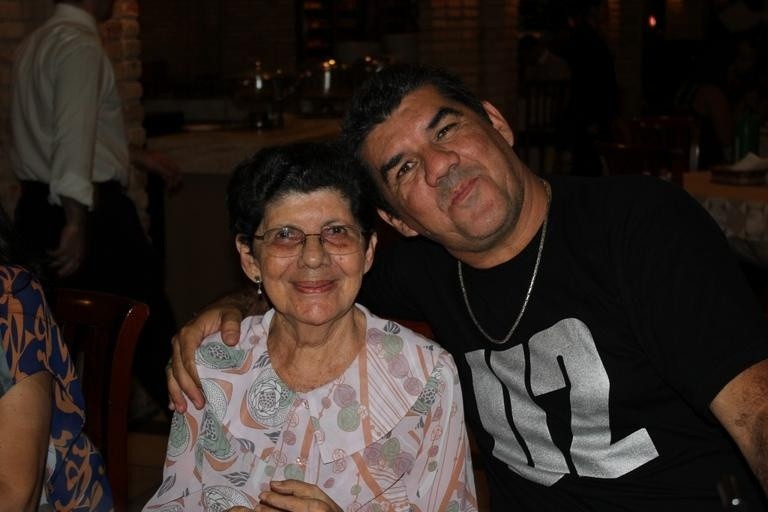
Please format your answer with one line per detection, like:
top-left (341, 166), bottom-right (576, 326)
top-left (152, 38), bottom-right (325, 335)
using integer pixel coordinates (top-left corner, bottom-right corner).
top-left (253, 225), bottom-right (364, 260)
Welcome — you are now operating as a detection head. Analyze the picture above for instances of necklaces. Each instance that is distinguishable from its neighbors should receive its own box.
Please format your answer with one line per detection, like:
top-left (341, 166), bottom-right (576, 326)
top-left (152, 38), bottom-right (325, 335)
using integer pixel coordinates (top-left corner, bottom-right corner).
top-left (455, 179), bottom-right (551, 345)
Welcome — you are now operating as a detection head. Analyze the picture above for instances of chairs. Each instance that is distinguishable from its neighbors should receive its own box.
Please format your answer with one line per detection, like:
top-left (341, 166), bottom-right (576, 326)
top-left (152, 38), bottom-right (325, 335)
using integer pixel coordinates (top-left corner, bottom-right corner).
top-left (34, 284), bottom-right (153, 510)
top-left (504, 77), bottom-right (575, 176)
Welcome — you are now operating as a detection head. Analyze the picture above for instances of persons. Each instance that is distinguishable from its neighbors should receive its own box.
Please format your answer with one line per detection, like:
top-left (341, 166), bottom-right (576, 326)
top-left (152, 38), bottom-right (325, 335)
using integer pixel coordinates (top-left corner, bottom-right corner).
top-left (138, 139), bottom-right (479, 511)
top-left (164, 60), bottom-right (768, 512)
top-left (10, 0), bottom-right (177, 420)
top-left (1, 263), bottom-right (121, 512)
top-left (553, 0), bottom-right (619, 173)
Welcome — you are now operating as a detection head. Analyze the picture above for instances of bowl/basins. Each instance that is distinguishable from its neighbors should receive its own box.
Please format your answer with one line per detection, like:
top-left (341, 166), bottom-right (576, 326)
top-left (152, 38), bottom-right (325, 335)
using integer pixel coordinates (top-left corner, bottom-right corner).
top-left (706, 164), bottom-right (768, 189)
